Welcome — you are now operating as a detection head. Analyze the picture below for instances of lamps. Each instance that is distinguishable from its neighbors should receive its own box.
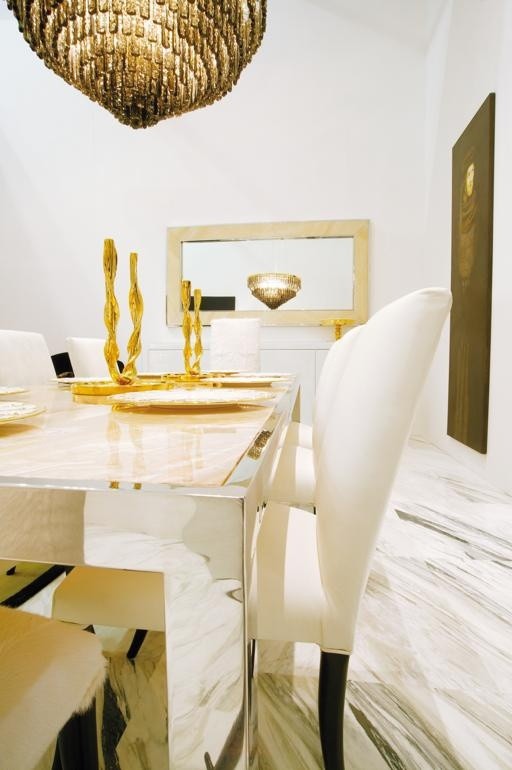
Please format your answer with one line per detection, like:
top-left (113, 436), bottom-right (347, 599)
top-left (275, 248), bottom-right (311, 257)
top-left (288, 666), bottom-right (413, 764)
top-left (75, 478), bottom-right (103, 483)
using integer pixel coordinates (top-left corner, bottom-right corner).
top-left (248, 274), bottom-right (301, 309)
top-left (7, 0), bottom-right (266, 129)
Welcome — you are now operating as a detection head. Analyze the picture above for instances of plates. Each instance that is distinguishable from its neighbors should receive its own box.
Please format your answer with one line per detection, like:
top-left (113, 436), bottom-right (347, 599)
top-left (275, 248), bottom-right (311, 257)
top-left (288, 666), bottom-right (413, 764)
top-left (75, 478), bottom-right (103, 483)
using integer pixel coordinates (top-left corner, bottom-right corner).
top-left (0, 400), bottom-right (49, 424)
top-left (107, 388), bottom-right (280, 406)
top-left (0, 385), bottom-right (33, 397)
top-left (50, 369), bottom-right (296, 386)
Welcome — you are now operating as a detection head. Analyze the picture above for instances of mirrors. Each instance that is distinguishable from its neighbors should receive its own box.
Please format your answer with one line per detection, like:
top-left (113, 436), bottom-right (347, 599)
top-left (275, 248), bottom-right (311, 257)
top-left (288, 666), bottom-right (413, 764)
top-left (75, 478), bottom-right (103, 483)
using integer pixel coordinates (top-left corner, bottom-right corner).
top-left (167, 219), bottom-right (368, 328)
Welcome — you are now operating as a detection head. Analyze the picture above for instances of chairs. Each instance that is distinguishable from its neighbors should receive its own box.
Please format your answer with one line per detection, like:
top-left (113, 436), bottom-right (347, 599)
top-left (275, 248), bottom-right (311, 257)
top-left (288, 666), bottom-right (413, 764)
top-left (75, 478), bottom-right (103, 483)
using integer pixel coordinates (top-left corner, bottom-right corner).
top-left (285, 421), bottom-right (312, 449)
top-left (260, 444), bottom-right (315, 512)
top-left (65, 337), bottom-right (109, 377)
top-left (51, 287), bottom-right (454, 770)
top-left (0, 605), bottom-right (107, 770)
top-left (0, 559), bottom-right (65, 609)
top-left (0, 330), bottom-right (56, 385)
